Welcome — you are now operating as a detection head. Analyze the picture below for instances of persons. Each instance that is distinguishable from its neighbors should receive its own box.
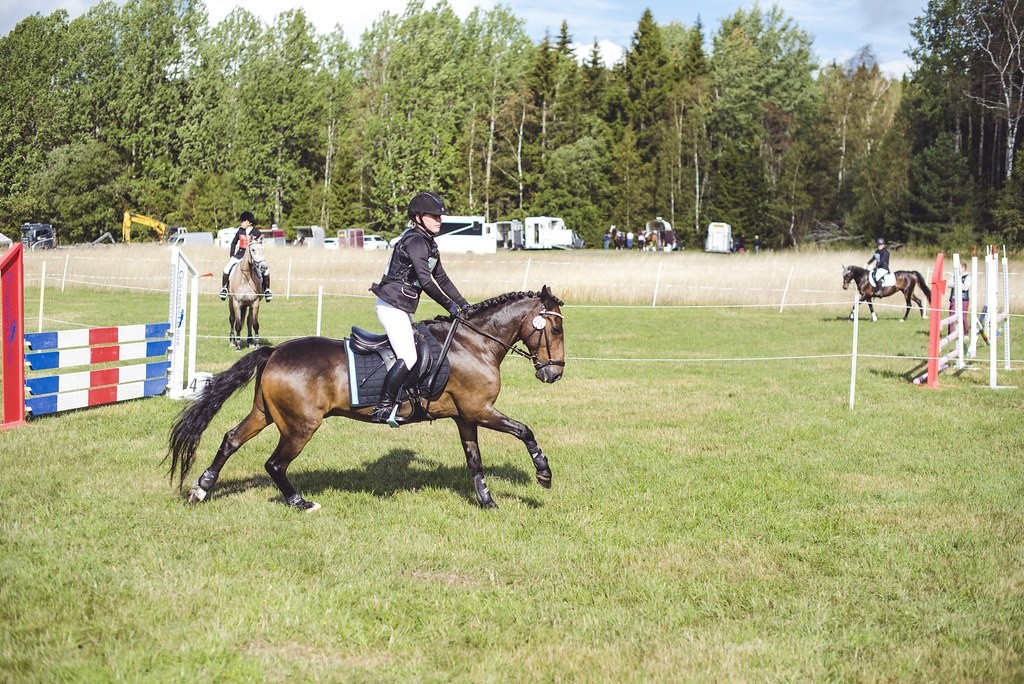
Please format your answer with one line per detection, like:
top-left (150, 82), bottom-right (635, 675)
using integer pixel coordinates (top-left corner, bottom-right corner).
top-left (603, 228), bottom-right (760, 254)
top-left (947, 263), bottom-right (970, 340)
top-left (864, 238), bottom-right (890, 298)
top-left (220, 210), bottom-right (271, 303)
top-left (366, 191), bottom-right (470, 427)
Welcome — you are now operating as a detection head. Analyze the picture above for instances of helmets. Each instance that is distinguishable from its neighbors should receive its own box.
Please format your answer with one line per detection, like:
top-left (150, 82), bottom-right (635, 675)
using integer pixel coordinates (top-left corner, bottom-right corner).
top-left (877, 237), bottom-right (886, 244)
top-left (237, 211), bottom-right (258, 225)
top-left (408, 191), bottom-right (447, 220)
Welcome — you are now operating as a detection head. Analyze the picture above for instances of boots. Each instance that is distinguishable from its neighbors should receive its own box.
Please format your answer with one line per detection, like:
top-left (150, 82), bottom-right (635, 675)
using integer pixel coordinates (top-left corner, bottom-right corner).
top-left (220, 272), bottom-right (229, 301)
top-left (874, 279), bottom-right (882, 298)
top-left (368, 358), bottom-right (410, 422)
top-left (263, 274), bottom-right (271, 302)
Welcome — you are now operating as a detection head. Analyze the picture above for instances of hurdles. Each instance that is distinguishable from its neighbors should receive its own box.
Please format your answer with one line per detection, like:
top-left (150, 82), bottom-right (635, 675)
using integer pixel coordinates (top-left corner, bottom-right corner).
top-left (913, 243), bottom-right (1024, 390)
top-left (0, 240), bottom-right (200, 431)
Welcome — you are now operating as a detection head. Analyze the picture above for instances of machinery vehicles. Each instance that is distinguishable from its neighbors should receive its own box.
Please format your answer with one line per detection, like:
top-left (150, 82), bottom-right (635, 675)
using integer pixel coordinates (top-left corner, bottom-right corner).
top-left (120, 209), bottom-right (189, 244)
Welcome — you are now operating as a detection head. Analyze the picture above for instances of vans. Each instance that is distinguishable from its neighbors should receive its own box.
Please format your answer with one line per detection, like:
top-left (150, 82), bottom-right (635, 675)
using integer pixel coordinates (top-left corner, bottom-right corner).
top-left (211, 214), bottom-right (577, 254)
top-left (164, 231), bottom-right (214, 245)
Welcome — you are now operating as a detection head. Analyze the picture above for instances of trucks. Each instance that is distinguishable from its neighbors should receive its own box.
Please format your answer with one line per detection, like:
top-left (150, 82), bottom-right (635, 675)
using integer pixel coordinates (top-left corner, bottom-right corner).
top-left (18, 221), bottom-right (59, 250)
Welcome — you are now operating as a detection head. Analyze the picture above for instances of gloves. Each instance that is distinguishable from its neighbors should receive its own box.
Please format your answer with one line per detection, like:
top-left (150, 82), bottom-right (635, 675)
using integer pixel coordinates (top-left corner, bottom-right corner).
top-left (461, 302), bottom-right (470, 311)
top-left (447, 301), bottom-right (459, 317)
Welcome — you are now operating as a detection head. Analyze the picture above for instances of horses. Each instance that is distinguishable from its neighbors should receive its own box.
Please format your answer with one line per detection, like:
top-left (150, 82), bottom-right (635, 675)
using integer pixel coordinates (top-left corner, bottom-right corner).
top-left (841, 264), bottom-right (931, 324)
top-left (226, 234), bottom-right (267, 353)
top-left (154, 285), bottom-right (565, 513)
top-left (608, 227), bottom-right (659, 251)
